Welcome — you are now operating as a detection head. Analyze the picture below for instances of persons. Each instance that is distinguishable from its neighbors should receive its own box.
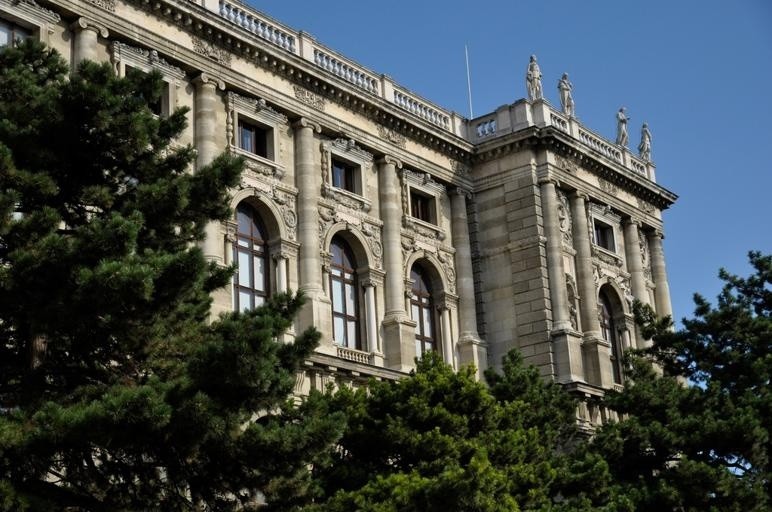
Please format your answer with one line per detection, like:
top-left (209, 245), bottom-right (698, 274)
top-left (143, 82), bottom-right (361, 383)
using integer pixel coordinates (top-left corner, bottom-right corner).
top-left (557, 72), bottom-right (575, 117)
top-left (616, 106), bottom-right (629, 147)
top-left (526, 54), bottom-right (544, 100)
top-left (638, 121), bottom-right (652, 162)
